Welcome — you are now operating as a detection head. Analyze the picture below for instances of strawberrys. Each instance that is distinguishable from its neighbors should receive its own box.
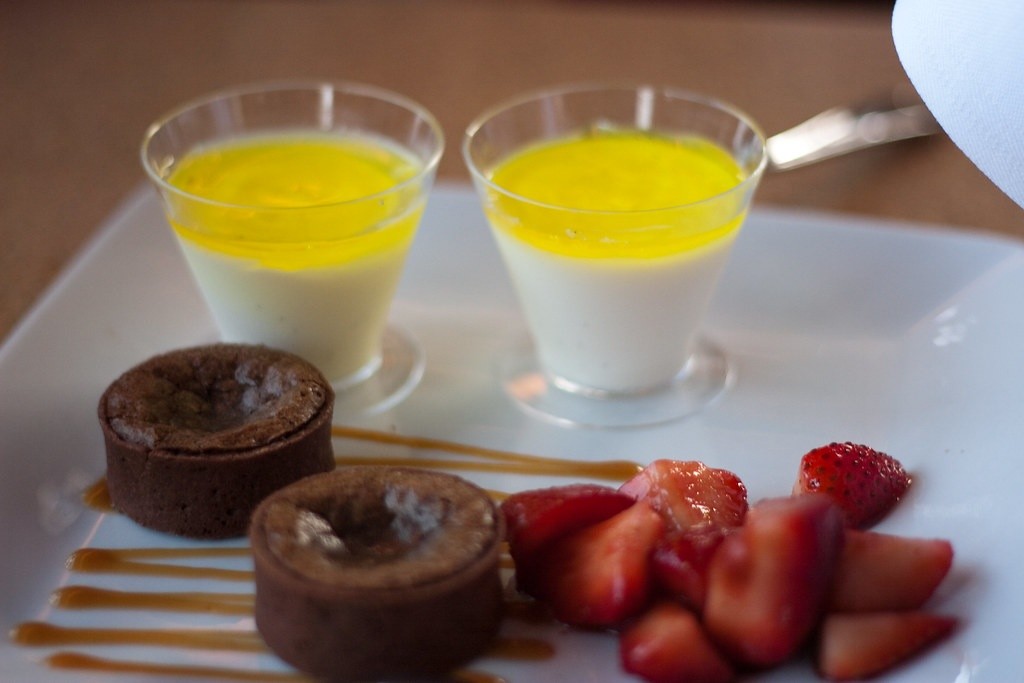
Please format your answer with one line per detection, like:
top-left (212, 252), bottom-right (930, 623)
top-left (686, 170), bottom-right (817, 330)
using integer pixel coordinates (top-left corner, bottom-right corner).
top-left (502, 441), bottom-right (961, 683)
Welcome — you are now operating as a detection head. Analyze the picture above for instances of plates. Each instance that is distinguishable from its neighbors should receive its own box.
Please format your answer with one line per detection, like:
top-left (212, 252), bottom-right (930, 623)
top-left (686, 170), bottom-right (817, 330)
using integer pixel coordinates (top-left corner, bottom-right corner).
top-left (1, 185), bottom-right (1023, 683)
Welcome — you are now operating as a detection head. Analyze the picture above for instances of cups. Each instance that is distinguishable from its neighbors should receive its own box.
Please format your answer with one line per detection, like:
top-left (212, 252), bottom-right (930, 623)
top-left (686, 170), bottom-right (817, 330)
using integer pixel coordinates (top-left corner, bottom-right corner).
top-left (462, 86), bottom-right (768, 426)
top-left (141, 83), bottom-right (446, 426)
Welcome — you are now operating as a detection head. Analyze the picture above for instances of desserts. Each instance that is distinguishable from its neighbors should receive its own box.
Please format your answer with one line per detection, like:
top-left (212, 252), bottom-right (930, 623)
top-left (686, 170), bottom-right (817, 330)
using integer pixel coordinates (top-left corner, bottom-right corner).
top-left (247, 465), bottom-right (508, 683)
top-left (95, 343), bottom-right (337, 539)
